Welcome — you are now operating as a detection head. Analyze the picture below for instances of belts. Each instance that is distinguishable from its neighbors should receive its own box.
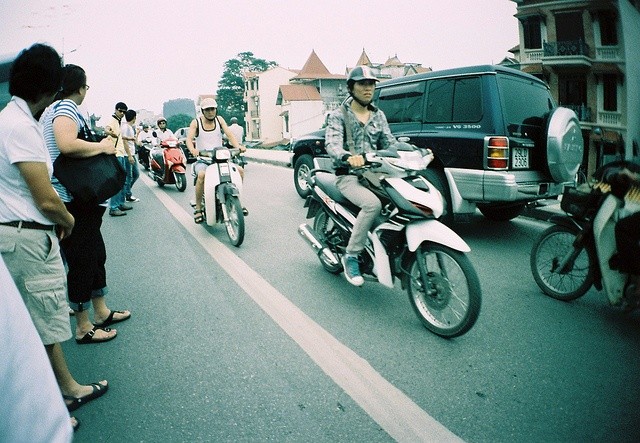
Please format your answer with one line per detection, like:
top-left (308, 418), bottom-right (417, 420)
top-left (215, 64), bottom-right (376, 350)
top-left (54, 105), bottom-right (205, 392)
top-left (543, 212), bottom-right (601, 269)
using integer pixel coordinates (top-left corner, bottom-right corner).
top-left (1, 219), bottom-right (53, 231)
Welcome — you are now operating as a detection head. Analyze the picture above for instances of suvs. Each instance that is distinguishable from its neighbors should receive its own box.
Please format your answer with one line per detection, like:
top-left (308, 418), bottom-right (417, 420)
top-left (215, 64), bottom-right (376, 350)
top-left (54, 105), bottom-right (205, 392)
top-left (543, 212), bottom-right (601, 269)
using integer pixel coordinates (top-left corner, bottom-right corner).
top-left (292, 63), bottom-right (584, 226)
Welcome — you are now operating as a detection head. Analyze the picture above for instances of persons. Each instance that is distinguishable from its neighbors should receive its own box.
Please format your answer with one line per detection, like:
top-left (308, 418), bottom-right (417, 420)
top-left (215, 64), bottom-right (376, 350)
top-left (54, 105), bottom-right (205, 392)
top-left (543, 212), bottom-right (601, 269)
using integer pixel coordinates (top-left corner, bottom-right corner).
top-left (616, 168), bottom-right (640, 313)
top-left (39, 63), bottom-right (131, 341)
top-left (324, 65), bottom-right (401, 284)
top-left (104, 101), bottom-right (133, 216)
top-left (1, 43), bottom-right (109, 430)
top-left (137, 123), bottom-right (154, 168)
top-left (223, 116), bottom-right (244, 148)
top-left (0, 253), bottom-right (74, 443)
top-left (186, 97), bottom-right (249, 223)
top-left (136, 121), bottom-right (143, 138)
top-left (123, 108), bottom-right (140, 201)
top-left (151, 116), bottom-right (184, 178)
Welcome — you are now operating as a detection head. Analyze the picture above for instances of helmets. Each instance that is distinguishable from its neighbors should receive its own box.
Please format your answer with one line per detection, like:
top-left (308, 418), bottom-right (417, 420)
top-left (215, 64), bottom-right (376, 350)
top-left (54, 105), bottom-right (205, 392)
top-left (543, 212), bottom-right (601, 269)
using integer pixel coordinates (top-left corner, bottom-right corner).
top-left (143, 124), bottom-right (148, 128)
top-left (346, 66), bottom-right (380, 83)
top-left (157, 117), bottom-right (167, 124)
top-left (200, 98), bottom-right (218, 110)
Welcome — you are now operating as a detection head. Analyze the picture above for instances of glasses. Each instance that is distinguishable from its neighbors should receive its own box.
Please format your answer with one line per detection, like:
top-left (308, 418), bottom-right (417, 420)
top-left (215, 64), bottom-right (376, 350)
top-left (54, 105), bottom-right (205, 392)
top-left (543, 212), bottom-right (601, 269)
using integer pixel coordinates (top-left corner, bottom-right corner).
top-left (118, 110), bottom-right (127, 114)
top-left (83, 84), bottom-right (90, 90)
top-left (205, 107), bottom-right (214, 111)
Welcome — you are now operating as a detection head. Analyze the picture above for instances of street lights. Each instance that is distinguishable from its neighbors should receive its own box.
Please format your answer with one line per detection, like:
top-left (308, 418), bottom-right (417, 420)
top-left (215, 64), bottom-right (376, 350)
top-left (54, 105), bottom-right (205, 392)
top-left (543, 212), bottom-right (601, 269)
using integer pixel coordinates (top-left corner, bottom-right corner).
top-left (60, 48), bottom-right (77, 67)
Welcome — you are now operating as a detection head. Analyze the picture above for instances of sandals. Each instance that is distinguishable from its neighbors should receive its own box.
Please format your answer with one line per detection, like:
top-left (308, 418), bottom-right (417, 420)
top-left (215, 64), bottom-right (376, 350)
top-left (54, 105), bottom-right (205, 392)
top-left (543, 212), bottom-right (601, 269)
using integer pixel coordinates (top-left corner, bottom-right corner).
top-left (63, 382), bottom-right (109, 411)
top-left (194, 210), bottom-right (203, 224)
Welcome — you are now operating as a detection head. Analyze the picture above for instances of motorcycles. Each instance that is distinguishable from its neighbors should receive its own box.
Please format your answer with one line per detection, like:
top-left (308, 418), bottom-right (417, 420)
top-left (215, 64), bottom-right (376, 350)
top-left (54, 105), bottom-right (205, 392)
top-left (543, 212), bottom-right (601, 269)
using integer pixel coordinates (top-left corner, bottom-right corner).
top-left (188, 145), bottom-right (249, 246)
top-left (293, 135), bottom-right (482, 338)
top-left (220, 137), bottom-right (248, 169)
top-left (137, 138), bottom-right (152, 170)
top-left (530, 125), bottom-right (640, 315)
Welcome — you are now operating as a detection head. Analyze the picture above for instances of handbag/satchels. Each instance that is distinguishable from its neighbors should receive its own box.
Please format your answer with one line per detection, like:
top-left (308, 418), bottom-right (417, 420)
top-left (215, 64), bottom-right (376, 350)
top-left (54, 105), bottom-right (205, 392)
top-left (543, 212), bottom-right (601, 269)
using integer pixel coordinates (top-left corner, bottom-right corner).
top-left (51, 100), bottom-right (126, 208)
top-left (340, 104), bottom-right (391, 201)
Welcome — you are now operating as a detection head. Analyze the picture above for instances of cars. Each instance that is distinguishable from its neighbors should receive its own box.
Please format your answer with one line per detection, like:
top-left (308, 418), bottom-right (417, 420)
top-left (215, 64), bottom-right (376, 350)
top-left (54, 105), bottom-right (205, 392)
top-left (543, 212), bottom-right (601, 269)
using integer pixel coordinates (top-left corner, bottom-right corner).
top-left (174, 126), bottom-right (198, 163)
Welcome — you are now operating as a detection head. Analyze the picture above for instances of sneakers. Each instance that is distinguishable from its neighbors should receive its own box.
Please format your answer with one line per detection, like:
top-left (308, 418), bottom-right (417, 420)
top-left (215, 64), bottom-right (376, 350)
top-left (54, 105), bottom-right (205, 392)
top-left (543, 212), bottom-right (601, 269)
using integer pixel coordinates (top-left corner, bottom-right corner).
top-left (341, 254), bottom-right (366, 288)
top-left (119, 204), bottom-right (134, 211)
top-left (110, 209), bottom-right (127, 217)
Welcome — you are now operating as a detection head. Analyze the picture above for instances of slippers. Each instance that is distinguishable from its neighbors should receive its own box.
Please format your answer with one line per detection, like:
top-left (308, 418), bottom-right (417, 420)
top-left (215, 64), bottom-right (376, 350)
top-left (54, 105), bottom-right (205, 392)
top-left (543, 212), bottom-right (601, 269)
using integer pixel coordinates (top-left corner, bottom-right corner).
top-left (93, 310), bottom-right (131, 328)
top-left (76, 326), bottom-right (117, 345)
